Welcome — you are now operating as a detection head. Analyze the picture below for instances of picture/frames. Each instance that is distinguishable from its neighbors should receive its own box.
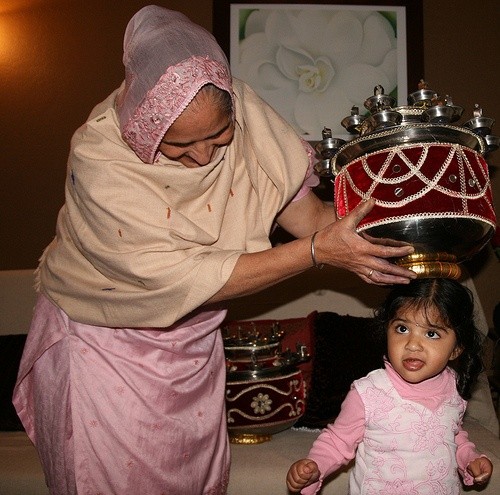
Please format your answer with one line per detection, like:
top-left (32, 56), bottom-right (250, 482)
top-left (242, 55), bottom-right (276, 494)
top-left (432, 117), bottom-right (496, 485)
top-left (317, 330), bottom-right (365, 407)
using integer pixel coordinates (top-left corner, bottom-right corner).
top-left (229, 4), bottom-right (408, 142)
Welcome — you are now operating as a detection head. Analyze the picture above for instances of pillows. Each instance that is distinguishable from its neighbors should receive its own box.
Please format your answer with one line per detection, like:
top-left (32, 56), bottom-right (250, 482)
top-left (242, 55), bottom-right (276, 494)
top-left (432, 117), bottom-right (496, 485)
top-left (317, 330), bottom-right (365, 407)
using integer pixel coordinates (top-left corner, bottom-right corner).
top-left (0, 334), bottom-right (28, 432)
top-left (221, 311), bottom-right (385, 433)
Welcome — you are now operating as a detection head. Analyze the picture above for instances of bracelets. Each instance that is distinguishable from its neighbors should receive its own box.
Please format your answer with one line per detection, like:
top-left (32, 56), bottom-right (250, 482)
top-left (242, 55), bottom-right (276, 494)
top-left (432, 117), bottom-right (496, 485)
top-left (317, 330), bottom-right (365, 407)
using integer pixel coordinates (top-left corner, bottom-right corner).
top-left (311, 231), bottom-right (325, 271)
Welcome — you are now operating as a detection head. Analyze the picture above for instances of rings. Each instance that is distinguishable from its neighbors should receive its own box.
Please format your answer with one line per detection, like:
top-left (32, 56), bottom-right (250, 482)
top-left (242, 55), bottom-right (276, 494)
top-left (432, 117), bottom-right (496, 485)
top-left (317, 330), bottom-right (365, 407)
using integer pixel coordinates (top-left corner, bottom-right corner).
top-left (367, 269), bottom-right (374, 279)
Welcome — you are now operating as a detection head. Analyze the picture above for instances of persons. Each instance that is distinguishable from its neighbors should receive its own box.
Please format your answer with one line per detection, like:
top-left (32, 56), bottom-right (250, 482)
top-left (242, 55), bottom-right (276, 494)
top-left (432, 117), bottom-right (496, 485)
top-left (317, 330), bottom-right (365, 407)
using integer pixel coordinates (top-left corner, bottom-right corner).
top-left (285, 275), bottom-right (494, 495)
top-left (11, 4), bottom-right (418, 495)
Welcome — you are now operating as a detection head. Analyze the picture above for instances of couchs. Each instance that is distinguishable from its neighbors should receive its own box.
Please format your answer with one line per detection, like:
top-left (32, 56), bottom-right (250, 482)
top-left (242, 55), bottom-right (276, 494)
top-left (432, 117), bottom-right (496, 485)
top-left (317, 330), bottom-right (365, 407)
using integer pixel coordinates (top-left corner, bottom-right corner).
top-left (0, 266), bottom-right (500, 495)
